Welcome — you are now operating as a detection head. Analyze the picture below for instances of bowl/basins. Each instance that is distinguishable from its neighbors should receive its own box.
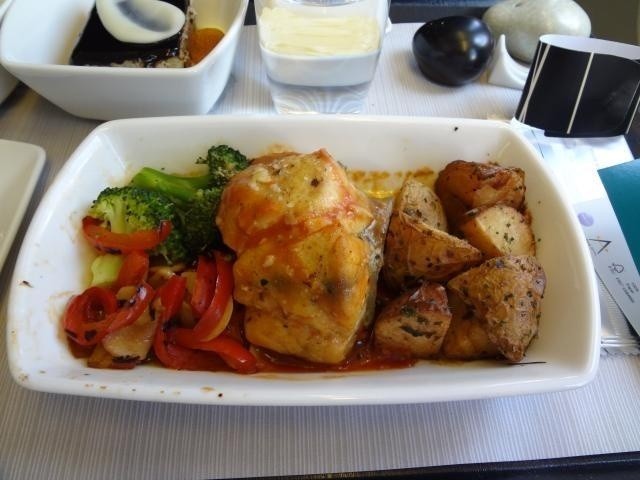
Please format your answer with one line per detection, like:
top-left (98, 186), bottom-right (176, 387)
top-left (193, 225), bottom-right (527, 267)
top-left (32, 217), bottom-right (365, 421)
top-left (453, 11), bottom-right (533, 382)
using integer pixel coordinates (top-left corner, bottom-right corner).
top-left (1, 3), bottom-right (250, 122)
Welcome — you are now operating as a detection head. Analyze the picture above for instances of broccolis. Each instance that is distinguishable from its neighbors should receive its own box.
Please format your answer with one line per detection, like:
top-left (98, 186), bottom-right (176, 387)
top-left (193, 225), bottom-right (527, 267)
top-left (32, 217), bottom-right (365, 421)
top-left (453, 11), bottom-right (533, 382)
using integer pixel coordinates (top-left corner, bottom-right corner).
top-left (84, 142), bottom-right (248, 265)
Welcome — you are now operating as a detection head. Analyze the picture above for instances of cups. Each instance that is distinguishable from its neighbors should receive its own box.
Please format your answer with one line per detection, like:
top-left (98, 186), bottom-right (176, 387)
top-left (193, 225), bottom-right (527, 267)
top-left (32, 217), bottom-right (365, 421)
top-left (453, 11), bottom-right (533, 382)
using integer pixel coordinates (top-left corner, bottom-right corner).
top-left (252, 0), bottom-right (390, 85)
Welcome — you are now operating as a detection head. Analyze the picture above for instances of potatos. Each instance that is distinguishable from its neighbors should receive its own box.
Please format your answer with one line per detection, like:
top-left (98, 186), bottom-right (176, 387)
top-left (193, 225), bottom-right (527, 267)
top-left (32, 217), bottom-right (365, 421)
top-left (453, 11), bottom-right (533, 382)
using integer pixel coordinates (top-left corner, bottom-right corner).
top-left (374, 161), bottom-right (546, 361)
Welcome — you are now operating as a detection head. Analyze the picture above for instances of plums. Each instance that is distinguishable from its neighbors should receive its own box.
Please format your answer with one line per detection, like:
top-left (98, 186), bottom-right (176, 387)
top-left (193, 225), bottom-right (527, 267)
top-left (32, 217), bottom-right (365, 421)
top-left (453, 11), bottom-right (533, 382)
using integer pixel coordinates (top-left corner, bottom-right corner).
top-left (412, 14), bottom-right (494, 88)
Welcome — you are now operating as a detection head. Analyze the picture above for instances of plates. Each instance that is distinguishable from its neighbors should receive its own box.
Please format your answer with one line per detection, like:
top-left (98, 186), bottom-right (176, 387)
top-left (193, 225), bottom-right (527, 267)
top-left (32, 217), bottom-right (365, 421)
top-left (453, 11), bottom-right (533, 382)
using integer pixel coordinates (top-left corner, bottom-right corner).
top-left (5, 115), bottom-right (598, 409)
top-left (2, 132), bottom-right (48, 268)
